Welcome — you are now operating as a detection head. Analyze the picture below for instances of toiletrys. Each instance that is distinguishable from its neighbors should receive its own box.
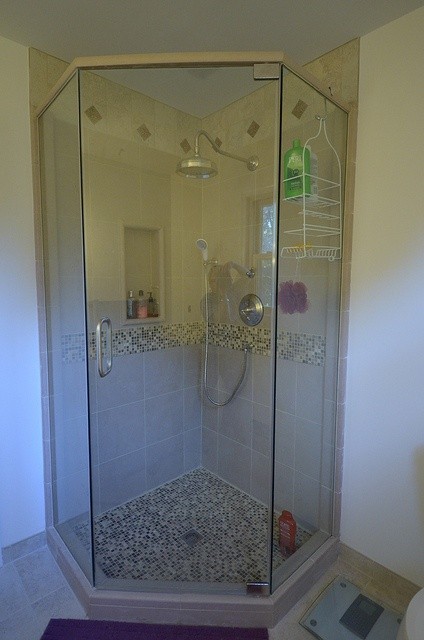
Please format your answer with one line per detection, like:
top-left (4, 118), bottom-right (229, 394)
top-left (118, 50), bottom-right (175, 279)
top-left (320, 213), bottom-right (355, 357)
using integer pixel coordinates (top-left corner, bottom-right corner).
top-left (278, 510), bottom-right (296, 557)
top-left (284, 138), bottom-right (311, 198)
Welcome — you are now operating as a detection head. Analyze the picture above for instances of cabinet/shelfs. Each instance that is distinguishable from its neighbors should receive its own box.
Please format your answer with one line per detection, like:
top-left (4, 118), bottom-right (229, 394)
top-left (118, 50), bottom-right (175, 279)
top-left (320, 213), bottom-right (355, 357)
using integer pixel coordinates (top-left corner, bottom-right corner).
top-left (279, 116), bottom-right (343, 263)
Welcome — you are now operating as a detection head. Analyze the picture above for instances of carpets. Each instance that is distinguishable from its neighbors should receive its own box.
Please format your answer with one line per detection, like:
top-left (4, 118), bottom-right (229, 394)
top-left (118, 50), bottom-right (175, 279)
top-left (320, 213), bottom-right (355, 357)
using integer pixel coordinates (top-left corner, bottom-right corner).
top-left (41, 618), bottom-right (270, 640)
